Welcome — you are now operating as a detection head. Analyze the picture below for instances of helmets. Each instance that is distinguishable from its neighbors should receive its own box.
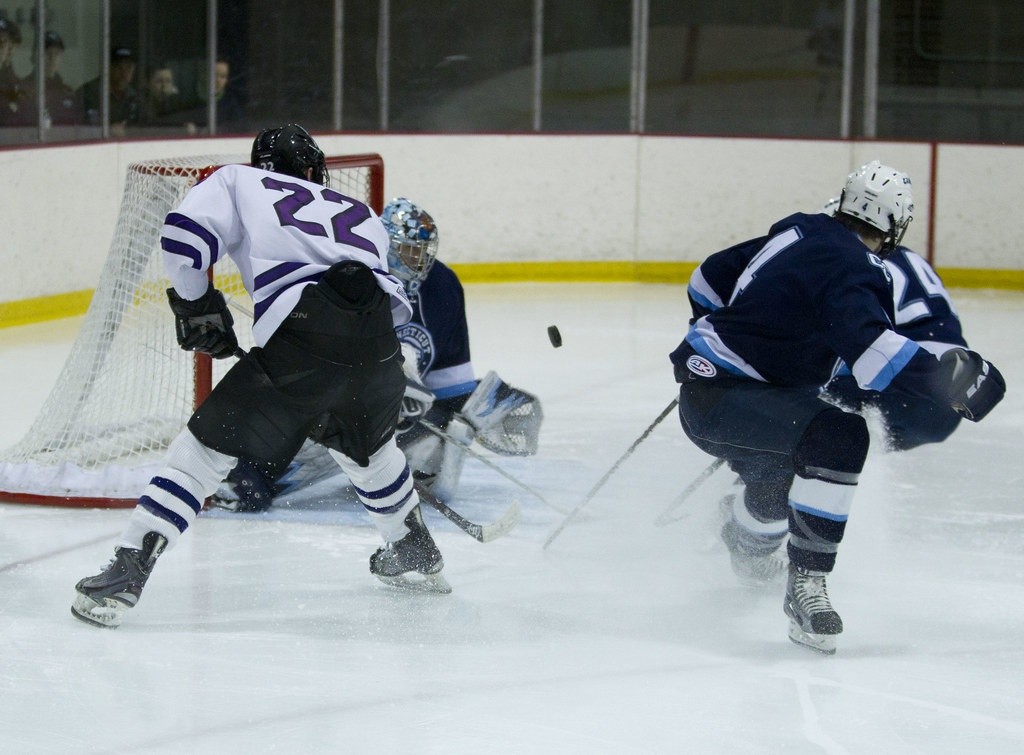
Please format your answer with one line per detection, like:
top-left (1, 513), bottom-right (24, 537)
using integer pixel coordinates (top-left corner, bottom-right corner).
top-left (839, 162), bottom-right (915, 259)
top-left (378, 198), bottom-right (439, 292)
top-left (250, 124), bottom-right (332, 187)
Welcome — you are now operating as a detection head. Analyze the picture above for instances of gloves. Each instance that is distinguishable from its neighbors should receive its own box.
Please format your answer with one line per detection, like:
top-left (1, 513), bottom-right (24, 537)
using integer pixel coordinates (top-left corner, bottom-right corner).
top-left (940, 345), bottom-right (1006, 423)
top-left (165, 286), bottom-right (238, 360)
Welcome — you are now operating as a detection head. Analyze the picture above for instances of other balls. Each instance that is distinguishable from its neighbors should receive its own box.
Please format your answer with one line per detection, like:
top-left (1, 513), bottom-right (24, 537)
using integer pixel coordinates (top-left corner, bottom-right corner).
top-left (548, 325), bottom-right (562, 347)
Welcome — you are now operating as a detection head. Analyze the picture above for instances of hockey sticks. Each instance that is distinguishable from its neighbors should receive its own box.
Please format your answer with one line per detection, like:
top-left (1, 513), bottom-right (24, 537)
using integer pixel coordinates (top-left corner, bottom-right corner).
top-left (233, 346), bottom-right (521, 543)
top-left (542, 394), bottom-right (680, 550)
top-left (416, 417), bottom-right (574, 516)
top-left (655, 455), bottom-right (727, 528)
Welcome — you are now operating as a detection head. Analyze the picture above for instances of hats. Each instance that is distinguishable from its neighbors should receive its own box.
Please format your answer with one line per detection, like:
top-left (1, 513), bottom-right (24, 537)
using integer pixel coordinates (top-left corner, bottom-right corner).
top-left (111, 43), bottom-right (136, 62)
top-left (42, 31), bottom-right (66, 52)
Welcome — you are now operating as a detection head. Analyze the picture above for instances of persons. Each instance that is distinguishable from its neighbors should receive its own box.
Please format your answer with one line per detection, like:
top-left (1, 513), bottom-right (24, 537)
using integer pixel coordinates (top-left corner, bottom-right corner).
top-left (669, 161), bottom-right (1005, 652)
top-left (0, 13), bottom-right (242, 134)
top-left (70, 122), bottom-right (543, 634)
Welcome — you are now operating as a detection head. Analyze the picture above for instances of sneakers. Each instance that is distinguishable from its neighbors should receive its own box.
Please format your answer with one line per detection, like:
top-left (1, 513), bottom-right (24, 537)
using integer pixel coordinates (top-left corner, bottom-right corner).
top-left (727, 552), bottom-right (790, 594)
top-left (370, 503), bottom-right (452, 595)
top-left (782, 567), bottom-right (844, 651)
top-left (71, 531), bottom-right (168, 631)
